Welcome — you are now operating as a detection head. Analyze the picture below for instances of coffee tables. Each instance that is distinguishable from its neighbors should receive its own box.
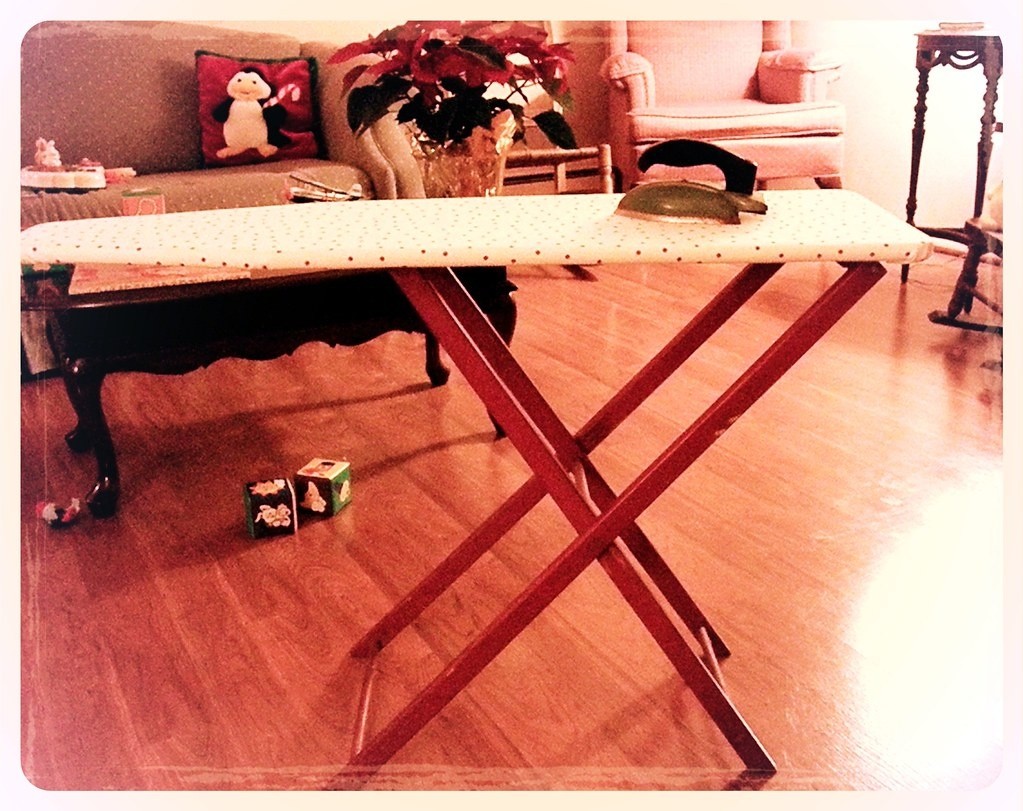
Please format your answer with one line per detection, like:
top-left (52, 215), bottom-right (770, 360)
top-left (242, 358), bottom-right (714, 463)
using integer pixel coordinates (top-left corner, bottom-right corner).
top-left (22, 264), bottom-right (520, 518)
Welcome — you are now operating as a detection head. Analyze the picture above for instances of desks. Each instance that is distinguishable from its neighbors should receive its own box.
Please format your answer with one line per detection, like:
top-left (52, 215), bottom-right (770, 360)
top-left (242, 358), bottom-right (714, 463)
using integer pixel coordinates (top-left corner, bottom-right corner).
top-left (44, 181), bottom-right (935, 783)
top-left (900, 26), bottom-right (1004, 311)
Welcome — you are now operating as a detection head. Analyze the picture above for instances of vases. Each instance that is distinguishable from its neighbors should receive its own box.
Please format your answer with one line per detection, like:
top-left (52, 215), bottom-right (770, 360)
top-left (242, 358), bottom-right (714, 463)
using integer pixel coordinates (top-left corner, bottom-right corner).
top-left (394, 103), bottom-right (517, 198)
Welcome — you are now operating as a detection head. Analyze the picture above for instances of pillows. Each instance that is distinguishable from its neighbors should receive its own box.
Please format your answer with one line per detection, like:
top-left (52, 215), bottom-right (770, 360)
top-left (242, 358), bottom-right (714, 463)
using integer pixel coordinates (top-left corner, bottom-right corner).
top-left (194, 50), bottom-right (320, 168)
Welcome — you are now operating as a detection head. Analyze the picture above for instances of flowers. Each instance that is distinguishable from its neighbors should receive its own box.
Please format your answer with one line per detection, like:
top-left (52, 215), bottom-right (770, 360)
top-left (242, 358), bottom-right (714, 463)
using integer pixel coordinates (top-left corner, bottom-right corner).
top-left (329, 19), bottom-right (581, 153)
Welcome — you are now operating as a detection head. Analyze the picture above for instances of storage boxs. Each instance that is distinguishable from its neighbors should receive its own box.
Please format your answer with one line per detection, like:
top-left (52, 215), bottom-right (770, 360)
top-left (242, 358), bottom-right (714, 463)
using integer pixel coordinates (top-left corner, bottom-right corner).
top-left (241, 478), bottom-right (298, 538)
top-left (294, 457), bottom-right (351, 517)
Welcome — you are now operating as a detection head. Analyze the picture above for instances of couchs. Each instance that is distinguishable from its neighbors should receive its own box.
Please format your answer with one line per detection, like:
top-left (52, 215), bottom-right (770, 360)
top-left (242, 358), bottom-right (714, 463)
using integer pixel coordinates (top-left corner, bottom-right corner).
top-left (600, 20), bottom-right (847, 191)
top-left (21, 20), bottom-right (427, 377)
